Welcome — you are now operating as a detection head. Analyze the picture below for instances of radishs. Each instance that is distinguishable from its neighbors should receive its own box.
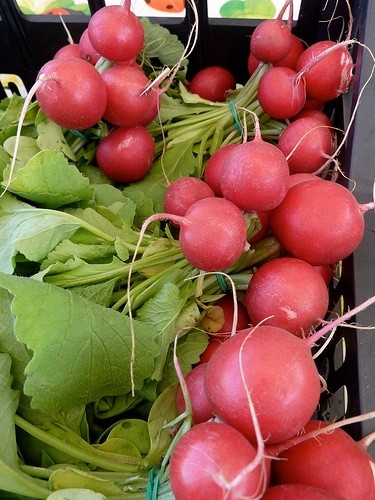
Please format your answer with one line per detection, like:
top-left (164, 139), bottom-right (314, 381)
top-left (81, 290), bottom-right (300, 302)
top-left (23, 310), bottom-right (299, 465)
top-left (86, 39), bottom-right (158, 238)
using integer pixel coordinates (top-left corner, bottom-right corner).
top-left (0, 0), bottom-right (375, 500)
